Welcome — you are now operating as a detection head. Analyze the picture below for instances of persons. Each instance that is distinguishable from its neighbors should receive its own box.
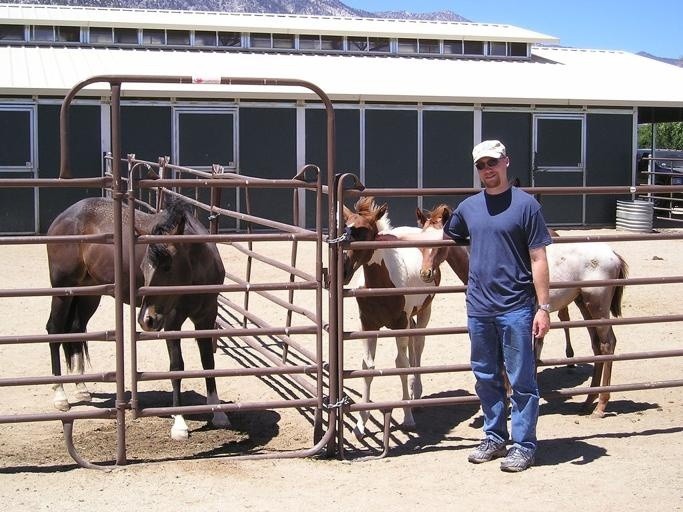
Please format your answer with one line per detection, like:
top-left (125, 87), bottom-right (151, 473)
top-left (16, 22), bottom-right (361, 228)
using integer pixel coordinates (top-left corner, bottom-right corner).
top-left (442, 141), bottom-right (552, 472)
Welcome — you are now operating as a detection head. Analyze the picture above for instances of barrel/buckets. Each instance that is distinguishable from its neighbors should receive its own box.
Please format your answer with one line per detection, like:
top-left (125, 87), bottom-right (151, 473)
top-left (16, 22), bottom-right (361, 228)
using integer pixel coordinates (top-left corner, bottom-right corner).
top-left (616, 200), bottom-right (654, 233)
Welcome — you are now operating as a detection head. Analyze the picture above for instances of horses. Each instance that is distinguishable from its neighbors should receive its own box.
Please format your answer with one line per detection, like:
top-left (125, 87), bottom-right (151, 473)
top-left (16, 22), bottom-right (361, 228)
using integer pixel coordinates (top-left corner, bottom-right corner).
top-left (538, 227), bottom-right (574, 359)
top-left (45, 195), bottom-right (231, 442)
top-left (341, 193), bottom-right (442, 441)
top-left (415, 202), bottom-right (630, 420)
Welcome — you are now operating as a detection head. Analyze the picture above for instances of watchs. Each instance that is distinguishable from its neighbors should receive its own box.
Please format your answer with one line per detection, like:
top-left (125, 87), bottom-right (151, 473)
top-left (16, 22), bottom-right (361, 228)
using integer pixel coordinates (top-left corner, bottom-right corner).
top-left (536, 303), bottom-right (552, 312)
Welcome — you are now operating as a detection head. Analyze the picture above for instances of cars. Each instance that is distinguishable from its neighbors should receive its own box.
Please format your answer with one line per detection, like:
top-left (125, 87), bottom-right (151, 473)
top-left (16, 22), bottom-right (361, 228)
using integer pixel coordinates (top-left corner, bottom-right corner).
top-left (636, 148), bottom-right (683, 215)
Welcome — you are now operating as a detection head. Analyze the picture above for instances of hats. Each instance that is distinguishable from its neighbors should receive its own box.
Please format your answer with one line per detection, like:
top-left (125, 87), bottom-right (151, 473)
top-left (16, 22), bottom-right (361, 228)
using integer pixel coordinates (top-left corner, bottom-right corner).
top-left (472, 139), bottom-right (507, 164)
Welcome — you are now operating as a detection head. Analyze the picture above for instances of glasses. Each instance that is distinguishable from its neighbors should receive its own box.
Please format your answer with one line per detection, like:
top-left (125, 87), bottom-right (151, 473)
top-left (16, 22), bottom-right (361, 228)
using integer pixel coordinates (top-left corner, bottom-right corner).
top-left (475, 157), bottom-right (505, 171)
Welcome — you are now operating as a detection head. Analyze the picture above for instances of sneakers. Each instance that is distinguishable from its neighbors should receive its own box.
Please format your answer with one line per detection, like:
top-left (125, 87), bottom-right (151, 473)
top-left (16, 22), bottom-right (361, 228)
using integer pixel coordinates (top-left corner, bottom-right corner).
top-left (500, 446), bottom-right (535, 472)
top-left (468, 437), bottom-right (507, 463)
